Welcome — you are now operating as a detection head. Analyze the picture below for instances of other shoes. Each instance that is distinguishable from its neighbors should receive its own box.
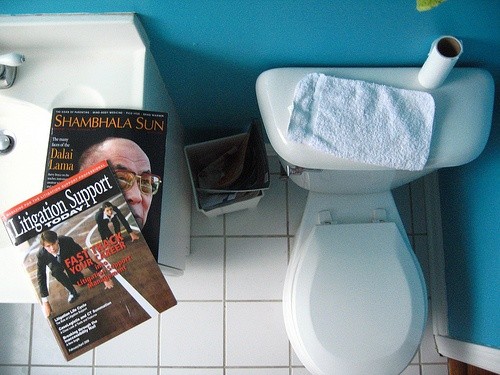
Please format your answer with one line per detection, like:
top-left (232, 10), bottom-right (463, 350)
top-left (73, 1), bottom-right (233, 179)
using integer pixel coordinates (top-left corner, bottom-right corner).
top-left (67, 291), bottom-right (77, 303)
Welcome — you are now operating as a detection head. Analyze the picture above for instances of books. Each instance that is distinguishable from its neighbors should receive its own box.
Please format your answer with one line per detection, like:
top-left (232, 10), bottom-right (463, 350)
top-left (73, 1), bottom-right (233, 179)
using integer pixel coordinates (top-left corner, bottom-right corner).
top-left (0, 159), bottom-right (179, 363)
top-left (195, 118), bottom-right (270, 210)
top-left (42, 107), bottom-right (169, 263)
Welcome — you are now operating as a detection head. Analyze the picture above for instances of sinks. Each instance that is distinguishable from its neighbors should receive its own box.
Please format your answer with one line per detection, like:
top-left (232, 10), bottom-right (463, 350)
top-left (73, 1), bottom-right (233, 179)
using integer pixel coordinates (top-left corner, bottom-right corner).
top-left (1, 94), bottom-right (52, 256)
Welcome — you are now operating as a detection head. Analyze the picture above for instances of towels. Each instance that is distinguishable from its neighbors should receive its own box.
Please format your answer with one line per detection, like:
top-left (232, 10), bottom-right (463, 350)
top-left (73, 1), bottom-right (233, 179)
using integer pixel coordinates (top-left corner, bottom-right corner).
top-left (283, 73), bottom-right (435, 171)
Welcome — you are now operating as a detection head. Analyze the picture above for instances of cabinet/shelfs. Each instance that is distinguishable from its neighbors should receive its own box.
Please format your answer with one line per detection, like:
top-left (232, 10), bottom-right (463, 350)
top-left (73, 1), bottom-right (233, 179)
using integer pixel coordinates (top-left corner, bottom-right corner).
top-left (144, 51), bottom-right (191, 277)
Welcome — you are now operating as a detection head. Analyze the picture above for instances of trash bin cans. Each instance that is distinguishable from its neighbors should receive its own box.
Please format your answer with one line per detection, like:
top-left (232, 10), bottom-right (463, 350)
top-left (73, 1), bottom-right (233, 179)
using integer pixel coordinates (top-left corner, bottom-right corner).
top-left (183, 134), bottom-right (265, 219)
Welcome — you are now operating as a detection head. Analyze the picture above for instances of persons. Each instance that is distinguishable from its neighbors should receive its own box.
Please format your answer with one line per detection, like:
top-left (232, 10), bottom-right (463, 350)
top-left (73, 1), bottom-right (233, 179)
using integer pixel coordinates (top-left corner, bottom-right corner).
top-left (94, 201), bottom-right (140, 252)
top-left (77, 137), bottom-right (153, 233)
top-left (36, 230), bottom-right (114, 318)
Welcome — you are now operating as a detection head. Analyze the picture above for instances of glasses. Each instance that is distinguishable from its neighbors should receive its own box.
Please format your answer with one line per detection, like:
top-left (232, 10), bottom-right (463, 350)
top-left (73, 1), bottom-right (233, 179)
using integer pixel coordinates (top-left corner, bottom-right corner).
top-left (114, 170), bottom-right (162, 195)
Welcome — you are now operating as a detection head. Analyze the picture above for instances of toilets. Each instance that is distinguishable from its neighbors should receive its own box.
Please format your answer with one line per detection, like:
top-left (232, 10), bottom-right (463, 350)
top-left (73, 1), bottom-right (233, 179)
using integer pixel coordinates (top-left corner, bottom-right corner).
top-left (256, 68), bottom-right (494, 375)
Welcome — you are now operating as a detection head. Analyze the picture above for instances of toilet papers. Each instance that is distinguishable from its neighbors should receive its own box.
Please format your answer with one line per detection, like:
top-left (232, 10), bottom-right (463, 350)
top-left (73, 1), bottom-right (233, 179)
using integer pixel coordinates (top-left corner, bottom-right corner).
top-left (418, 36), bottom-right (463, 89)
